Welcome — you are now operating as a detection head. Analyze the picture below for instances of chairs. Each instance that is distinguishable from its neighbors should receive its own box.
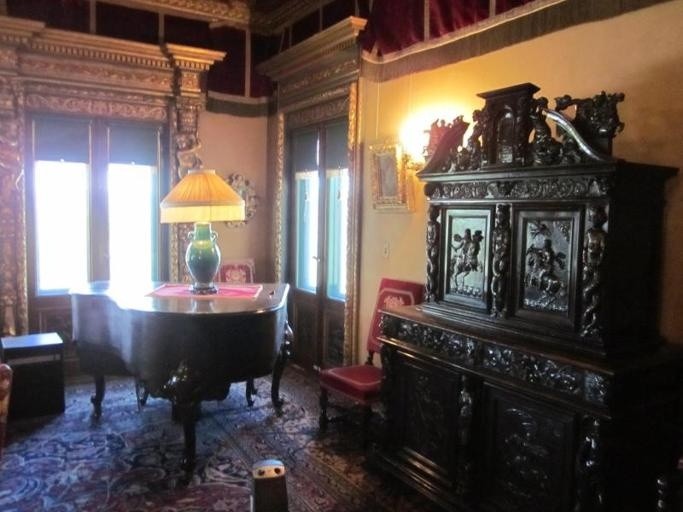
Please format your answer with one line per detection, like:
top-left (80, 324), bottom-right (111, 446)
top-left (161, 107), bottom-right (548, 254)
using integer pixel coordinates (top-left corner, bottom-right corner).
top-left (216, 258), bottom-right (257, 284)
top-left (317, 279), bottom-right (427, 447)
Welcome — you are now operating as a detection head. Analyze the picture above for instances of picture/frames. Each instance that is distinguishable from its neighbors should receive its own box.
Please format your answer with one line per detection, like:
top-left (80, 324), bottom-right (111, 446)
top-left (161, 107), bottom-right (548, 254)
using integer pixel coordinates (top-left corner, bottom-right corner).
top-left (369, 142), bottom-right (404, 204)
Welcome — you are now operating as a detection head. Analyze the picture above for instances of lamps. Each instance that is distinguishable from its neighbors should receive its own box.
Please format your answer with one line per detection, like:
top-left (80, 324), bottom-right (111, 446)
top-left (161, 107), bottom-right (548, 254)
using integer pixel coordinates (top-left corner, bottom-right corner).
top-left (159, 166), bottom-right (246, 296)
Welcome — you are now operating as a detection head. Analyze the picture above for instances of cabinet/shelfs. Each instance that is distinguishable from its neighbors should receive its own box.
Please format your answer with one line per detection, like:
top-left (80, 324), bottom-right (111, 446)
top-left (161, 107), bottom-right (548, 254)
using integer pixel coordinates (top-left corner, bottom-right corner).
top-left (359, 81), bottom-right (683, 512)
top-left (0, 330), bottom-right (66, 419)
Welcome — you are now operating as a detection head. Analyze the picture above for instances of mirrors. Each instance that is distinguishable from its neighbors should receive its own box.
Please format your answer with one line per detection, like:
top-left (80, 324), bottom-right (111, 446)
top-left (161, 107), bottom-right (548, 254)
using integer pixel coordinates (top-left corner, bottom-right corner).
top-left (221, 171), bottom-right (258, 227)
